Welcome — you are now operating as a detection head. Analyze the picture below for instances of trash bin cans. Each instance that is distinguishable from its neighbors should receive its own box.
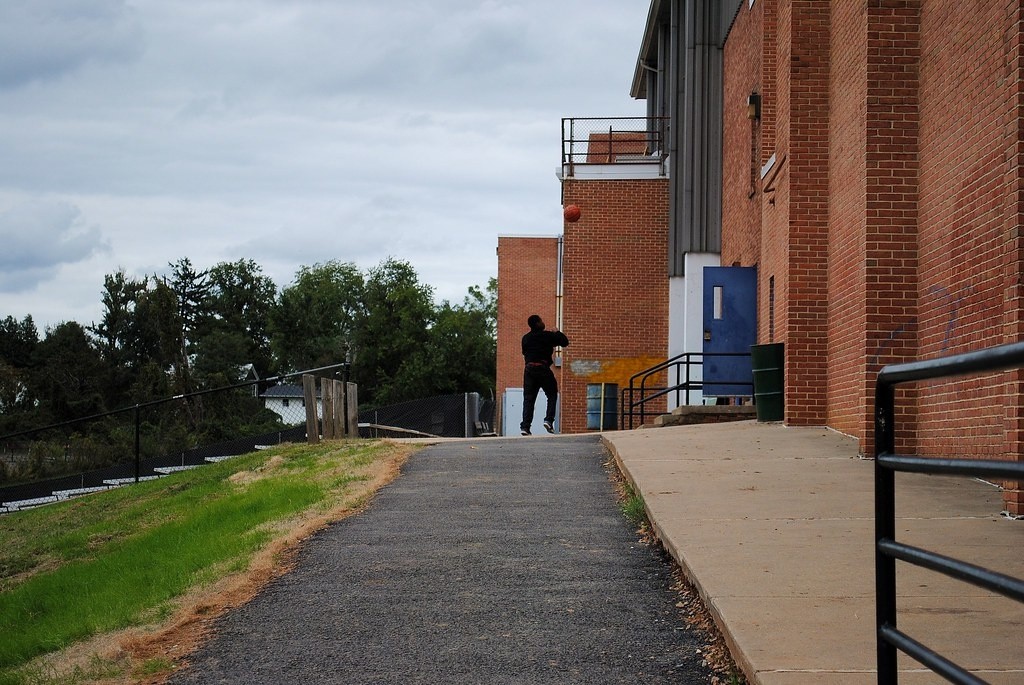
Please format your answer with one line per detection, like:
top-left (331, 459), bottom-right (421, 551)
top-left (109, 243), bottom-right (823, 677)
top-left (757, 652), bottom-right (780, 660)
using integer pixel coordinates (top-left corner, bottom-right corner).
top-left (748, 342), bottom-right (784, 423)
top-left (586, 383), bottom-right (619, 431)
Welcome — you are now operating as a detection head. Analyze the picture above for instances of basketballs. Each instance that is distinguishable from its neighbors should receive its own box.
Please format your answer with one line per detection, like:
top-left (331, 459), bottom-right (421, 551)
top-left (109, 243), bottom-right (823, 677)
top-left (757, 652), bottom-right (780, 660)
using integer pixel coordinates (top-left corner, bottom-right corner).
top-left (564, 205), bottom-right (581, 223)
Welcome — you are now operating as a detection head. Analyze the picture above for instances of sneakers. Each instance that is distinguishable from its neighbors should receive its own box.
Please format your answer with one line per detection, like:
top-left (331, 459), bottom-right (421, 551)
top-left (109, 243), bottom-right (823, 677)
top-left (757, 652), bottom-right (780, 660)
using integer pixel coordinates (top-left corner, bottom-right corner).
top-left (543, 421), bottom-right (555, 433)
top-left (520, 428), bottom-right (533, 436)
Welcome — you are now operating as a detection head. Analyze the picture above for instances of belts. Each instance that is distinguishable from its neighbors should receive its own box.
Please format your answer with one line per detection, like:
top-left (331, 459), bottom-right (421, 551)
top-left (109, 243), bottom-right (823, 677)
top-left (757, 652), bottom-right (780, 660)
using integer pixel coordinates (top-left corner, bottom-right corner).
top-left (528, 361), bottom-right (541, 366)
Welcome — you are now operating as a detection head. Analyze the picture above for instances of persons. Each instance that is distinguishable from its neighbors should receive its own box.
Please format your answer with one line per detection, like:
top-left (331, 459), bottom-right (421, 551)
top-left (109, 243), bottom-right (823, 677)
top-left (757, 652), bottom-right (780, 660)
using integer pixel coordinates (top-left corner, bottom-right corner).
top-left (520, 315), bottom-right (569, 436)
